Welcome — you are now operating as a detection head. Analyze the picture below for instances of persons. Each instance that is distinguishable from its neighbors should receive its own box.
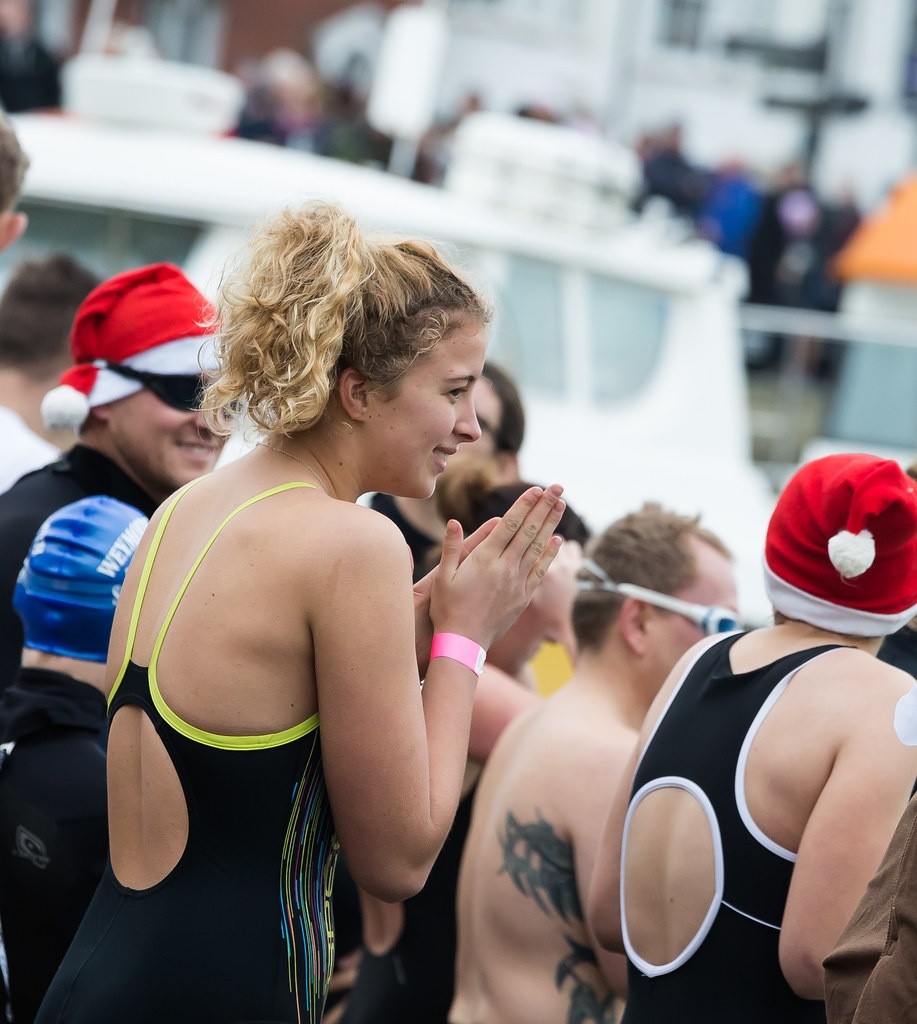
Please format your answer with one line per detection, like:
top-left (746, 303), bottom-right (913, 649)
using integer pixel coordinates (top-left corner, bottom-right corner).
top-left (0, 117), bottom-right (566, 1024)
top-left (0, 0), bottom-right (61, 113)
top-left (235, 45), bottom-right (861, 392)
top-left (447, 452), bottom-right (917, 1024)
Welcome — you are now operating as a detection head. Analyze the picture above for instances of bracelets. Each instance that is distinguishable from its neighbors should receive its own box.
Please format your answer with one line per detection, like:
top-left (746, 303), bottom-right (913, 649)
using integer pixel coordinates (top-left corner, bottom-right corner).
top-left (429, 632), bottom-right (487, 677)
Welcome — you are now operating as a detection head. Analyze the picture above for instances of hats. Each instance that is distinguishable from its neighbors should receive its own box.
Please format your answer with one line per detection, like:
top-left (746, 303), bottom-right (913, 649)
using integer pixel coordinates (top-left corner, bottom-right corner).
top-left (39, 264), bottom-right (224, 434)
top-left (765, 453), bottom-right (917, 636)
top-left (13, 496), bottom-right (151, 663)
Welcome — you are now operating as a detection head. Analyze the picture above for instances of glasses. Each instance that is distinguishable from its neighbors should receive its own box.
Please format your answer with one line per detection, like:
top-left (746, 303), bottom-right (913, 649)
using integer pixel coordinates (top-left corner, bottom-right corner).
top-left (101, 360), bottom-right (207, 413)
top-left (613, 582), bottom-right (742, 636)
